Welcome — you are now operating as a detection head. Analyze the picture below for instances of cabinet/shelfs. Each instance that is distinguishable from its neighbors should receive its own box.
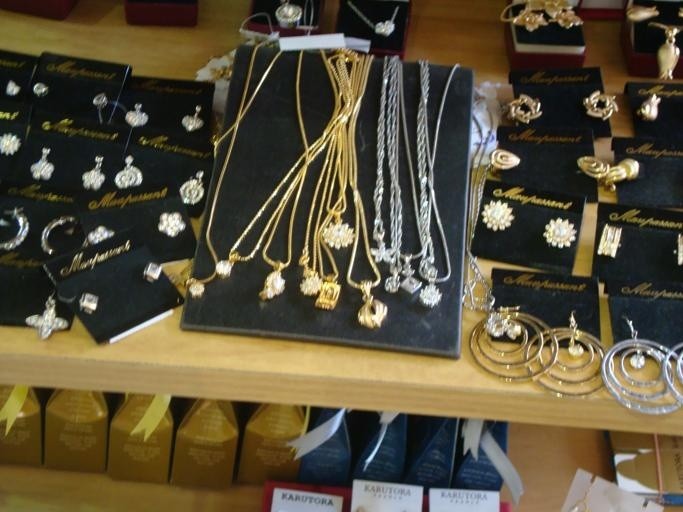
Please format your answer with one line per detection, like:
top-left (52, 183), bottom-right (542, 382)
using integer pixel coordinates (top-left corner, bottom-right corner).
top-left (0, 0), bottom-right (683, 512)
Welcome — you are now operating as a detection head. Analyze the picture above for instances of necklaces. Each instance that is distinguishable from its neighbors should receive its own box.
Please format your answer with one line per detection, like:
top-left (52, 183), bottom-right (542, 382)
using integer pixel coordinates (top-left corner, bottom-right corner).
top-left (348, 1), bottom-right (401, 35)
top-left (652, 23), bottom-right (683, 79)
top-left (371, 56), bottom-right (459, 307)
top-left (275, 1), bottom-right (302, 26)
top-left (462, 95), bottom-right (520, 311)
top-left (190, 41), bottom-right (387, 327)
